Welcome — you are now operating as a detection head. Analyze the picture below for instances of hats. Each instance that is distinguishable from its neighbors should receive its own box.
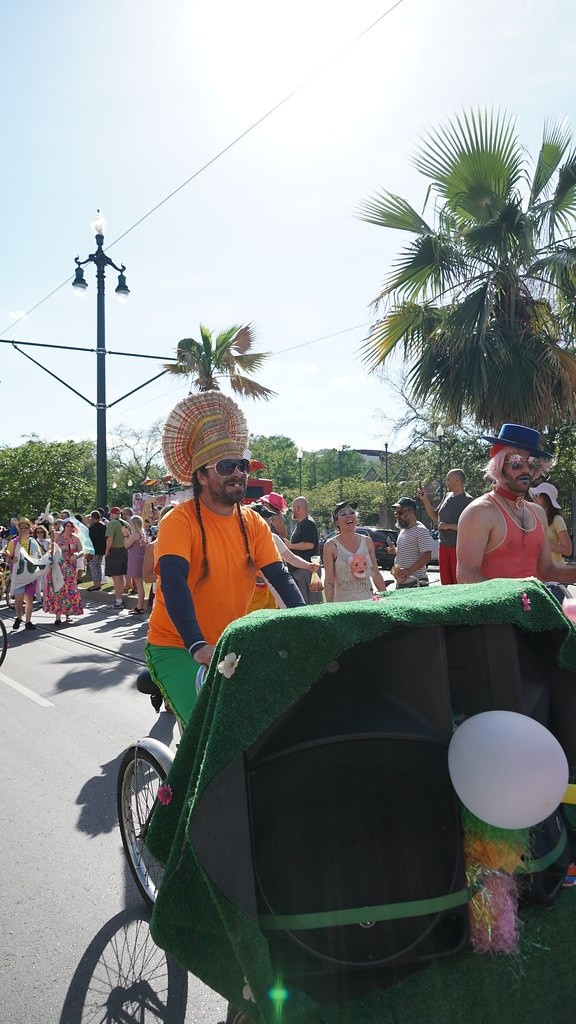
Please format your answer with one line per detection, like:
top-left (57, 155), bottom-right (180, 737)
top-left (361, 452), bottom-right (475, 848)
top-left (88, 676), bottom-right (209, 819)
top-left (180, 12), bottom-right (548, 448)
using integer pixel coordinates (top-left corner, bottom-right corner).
top-left (391, 497), bottom-right (417, 509)
top-left (110, 507), bottom-right (121, 514)
top-left (260, 492), bottom-right (283, 510)
top-left (161, 391), bottom-right (248, 486)
top-left (528, 482), bottom-right (562, 509)
top-left (16, 518), bottom-right (33, 530)
top-left (251, 505), bottom-right (276, 518)
top-left (480, 424), bottom-right (553, 458)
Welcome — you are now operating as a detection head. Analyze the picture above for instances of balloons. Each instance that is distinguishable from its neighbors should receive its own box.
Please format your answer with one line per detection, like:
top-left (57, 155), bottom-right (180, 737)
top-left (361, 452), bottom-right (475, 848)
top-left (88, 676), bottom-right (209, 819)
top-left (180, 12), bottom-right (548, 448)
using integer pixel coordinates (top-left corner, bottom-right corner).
top-left (448, 709), bottom-right (569, 831)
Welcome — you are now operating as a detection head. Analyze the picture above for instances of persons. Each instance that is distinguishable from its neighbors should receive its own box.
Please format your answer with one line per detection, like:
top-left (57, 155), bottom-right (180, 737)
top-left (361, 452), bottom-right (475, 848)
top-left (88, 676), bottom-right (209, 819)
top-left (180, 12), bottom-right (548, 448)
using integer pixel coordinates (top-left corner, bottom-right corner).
top-left (323, 500), bottom-right (387, 603)
top-left (526, 482), bottom-right (570, 568)
top-left (145, 501), bottom-right (179, 610)
top-left (144, 445), bottom-right (307, 734)
top-left (46, 523), bottom-right (84, 624)
top-left (3, 519), bottom-right (43, 629)
top-left (454, 424), bottom-right (576, 587)
top-left (249, 503), bottom-right (320, 572)
top-left (11, 507), bottom-right (158, 614)
top-left (287, 497), bottom-right (322, 605)
top-left (418, 469), bottom-right (472, 584)
top-left (386, 496), bottom-right (432, 589)
top-left (256, 492), bottom-right (286, 539)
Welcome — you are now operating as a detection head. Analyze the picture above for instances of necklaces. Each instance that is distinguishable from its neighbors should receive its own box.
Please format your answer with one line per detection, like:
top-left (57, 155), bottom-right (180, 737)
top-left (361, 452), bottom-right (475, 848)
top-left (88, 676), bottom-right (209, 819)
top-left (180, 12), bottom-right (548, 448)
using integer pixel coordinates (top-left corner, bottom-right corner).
top-left (494, 486), bottom-right (526, 527)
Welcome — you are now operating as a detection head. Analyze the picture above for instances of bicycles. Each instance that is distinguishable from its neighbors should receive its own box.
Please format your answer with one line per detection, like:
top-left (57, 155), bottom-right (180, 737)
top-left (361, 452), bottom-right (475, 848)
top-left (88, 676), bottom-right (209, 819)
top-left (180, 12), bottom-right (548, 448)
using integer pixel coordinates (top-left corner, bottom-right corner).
top-left (0, 552), bottom-right (16, 609)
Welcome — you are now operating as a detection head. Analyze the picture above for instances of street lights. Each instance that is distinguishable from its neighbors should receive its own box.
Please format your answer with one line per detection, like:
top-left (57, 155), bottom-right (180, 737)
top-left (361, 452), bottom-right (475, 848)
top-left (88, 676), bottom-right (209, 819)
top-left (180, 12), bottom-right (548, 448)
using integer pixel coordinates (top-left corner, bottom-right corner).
top-left (71, 207), bottom-right (130, 519)
top-left (127, 479), bottom-right (133, 507)
top-left (296, 448), bottom-right (304, 496)
top-left (112, 482), bottom-right (117, 508)
top-left (382, 433), bottom-right (391, 486)
top-left (336, 441), bottom-right (344, 503)
top-left (435, 424), bottom-right (445, 500)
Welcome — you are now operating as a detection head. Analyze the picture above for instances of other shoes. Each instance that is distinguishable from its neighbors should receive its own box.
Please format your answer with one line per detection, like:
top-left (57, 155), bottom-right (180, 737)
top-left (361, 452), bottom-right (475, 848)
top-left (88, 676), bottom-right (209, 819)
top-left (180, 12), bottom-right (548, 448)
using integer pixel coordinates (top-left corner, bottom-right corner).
top-left (25, 622), bottom-right (35, 630)
top-left (55, 620), bottom-right (61, 625)
top-left (87, 582), bottom-right (139, 595)
top-left (66, 617), bottom-right (73, 622)
top-left (109, 602), bottom-right (125, 608)
top-left (13, 618), bottom-right (22, 629)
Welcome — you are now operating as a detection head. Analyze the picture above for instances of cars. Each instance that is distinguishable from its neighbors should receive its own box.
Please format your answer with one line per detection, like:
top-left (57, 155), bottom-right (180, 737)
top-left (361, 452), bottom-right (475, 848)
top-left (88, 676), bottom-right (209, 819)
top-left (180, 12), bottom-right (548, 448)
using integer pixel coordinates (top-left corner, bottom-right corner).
top-left (424, 530), bottom-right (440, 569)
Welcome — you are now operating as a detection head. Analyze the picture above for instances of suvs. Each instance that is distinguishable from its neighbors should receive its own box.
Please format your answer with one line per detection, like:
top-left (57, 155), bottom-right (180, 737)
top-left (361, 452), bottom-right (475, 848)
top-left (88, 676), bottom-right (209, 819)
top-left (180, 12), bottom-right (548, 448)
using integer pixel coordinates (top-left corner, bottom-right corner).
top-left (319, 526), bottom-right (398, 570)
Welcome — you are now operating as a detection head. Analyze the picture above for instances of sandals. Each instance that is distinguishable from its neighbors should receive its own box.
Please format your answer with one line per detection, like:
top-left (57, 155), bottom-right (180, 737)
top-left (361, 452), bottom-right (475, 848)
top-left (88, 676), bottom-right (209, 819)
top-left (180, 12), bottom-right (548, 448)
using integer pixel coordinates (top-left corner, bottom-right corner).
top-left (129, 607), bottom-right (144, 614)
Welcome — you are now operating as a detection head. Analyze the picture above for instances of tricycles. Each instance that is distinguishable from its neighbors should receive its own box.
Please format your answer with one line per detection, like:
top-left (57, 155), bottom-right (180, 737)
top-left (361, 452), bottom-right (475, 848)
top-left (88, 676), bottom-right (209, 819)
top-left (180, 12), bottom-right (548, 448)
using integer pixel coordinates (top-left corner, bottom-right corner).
top-left (116, 576), bottom-right (575, 1023)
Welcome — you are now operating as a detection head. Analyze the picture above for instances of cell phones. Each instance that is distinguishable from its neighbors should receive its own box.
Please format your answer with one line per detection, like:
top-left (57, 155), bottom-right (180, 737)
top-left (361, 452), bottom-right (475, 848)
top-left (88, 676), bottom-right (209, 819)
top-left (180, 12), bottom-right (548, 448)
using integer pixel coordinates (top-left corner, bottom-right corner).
top-left (419, 481), bottom-right (423, 496)
top-left (151, 503), bottom-right (154, 513)
top-left (387, 537), bottom-right (394, 548)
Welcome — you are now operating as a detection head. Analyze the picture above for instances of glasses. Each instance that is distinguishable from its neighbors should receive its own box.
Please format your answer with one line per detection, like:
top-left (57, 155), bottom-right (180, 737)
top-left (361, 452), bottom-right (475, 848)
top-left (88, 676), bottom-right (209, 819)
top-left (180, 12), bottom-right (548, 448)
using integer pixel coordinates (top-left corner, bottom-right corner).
top-left (393, 509), bottom-right (413, 516)
top-left (36, 531), bottom-right (44, 533)
top-left (533, 493), bottom-right (546, 497)
top-left (504, 454), bottom-right (539, 468)
top-left (205, 459), bottom-right (251, 478)
